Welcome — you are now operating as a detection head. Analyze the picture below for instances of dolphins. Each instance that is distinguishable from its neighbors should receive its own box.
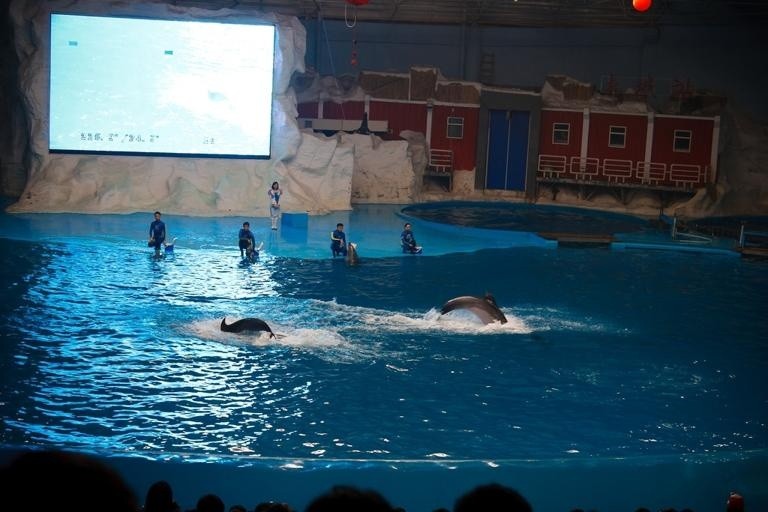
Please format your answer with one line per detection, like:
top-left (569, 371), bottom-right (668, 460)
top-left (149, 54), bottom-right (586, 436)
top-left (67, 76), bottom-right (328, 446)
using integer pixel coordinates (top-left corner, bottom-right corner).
top-left (437, 293), bottom-right (509, 327)
top-left (220, 316), bottom-right (277, 340)
top-left (348, 241), bottom-right (367, 264)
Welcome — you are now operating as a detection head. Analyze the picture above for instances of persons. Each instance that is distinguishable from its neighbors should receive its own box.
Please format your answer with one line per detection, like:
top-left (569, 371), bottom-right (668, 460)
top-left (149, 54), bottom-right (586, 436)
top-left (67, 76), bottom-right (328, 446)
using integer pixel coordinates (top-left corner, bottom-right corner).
top-left (399, 222), bottom-right (417, 255)
top-left (237, 220), bottom-right (256, 259)
top-left (266, 180), bottom-right (282, 230)
top-left (0, 448), bottom-right (746, 512)
top-left (147, 209), bottom-right (166, 250)
top-left (328, 221), bottom-right (348, 258)
